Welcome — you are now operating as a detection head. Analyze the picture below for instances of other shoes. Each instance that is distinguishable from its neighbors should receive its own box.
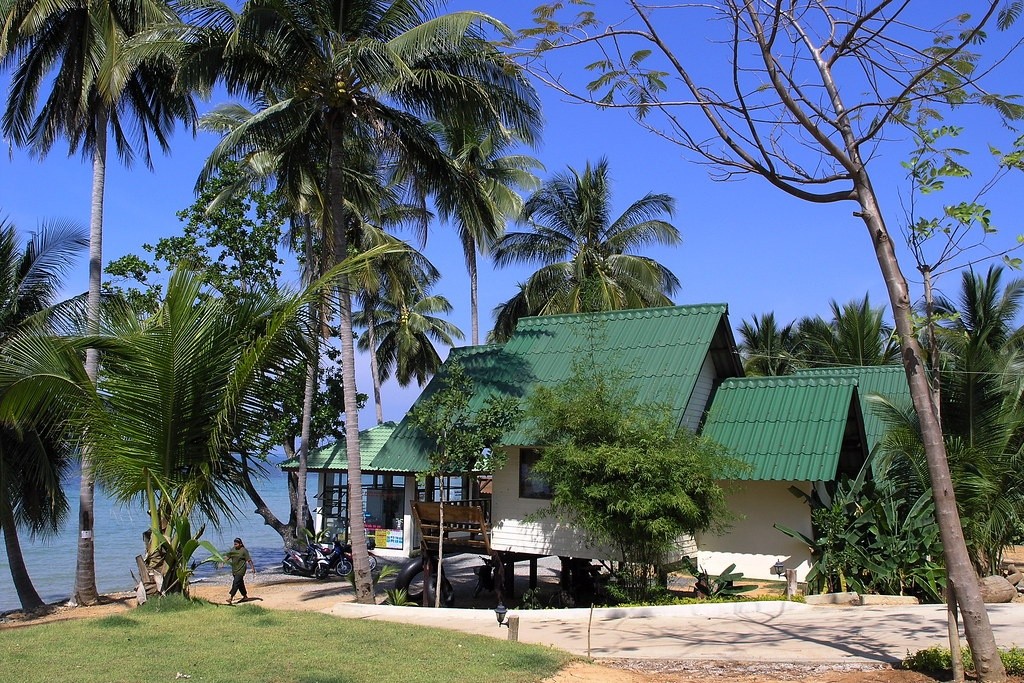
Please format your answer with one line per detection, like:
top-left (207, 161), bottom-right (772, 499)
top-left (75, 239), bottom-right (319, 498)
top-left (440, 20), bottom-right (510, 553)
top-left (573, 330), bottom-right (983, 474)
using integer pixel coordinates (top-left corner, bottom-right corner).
top-left (241, 596), bottom-right (249, 602)
top-left (225, 598), bottom-right (232, 604)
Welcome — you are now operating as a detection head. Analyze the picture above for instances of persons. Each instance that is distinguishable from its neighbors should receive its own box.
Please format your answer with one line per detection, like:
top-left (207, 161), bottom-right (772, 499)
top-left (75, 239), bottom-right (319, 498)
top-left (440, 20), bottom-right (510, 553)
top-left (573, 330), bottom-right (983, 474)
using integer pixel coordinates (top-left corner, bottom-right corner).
top-left (218, 536), bottom-right (256, 604)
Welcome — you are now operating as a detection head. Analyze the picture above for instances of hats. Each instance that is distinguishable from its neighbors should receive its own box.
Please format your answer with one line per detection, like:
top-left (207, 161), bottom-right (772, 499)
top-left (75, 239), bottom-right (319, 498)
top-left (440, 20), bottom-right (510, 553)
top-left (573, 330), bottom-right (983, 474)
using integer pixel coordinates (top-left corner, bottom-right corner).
top-left (233, 537), bottom-right (242, 544)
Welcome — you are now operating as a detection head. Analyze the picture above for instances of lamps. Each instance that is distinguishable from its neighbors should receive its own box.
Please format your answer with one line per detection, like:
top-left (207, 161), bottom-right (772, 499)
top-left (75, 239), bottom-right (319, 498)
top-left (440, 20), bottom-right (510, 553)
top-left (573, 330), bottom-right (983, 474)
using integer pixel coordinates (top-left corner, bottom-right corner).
top-left (494, 602), bottom-right (510, 629)
top-left (773, 559), bottom-right (788, 579)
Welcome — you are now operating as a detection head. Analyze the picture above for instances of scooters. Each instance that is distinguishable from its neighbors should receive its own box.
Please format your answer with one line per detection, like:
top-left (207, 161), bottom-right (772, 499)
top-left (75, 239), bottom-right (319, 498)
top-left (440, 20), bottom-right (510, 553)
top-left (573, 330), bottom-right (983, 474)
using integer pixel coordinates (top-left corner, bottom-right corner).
top-left (281, 533), bottom-right (377, 580)
